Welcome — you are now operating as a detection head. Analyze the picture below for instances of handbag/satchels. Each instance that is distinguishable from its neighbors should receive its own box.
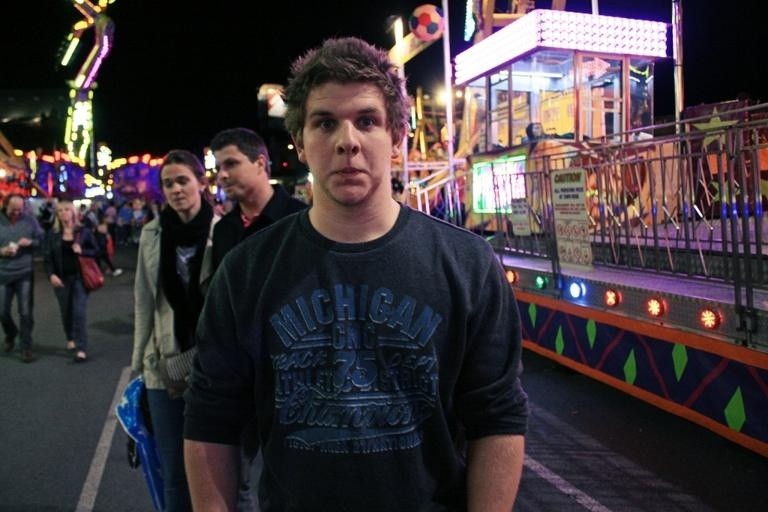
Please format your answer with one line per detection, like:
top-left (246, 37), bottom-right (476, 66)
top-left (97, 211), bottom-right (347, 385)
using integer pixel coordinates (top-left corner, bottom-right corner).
top-left (116, 372), bottom-right (164, 512)
top-left (74, 233), bottom-right (105, 291)
top-left (157, 346), bottom-right (197, 402)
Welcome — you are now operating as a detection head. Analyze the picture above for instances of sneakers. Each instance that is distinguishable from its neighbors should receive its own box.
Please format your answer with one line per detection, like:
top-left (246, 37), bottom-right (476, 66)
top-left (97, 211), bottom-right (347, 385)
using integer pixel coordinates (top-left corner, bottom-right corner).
top-left (2, 327), bottom-right (88, 364)
top-left (113, 268), bottom-right (123, 277)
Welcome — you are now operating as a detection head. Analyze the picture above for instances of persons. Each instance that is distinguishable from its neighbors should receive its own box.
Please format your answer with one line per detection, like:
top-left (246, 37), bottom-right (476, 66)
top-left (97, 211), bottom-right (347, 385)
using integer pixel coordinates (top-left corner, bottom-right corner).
top-left (213, 129), bottom-right (310, 279)
top-left (42, 200), bottom-right (101, 362)
top-left (1, 192), bottom-right (48, 364)
top-left (130, 151), bottom-right (226, 511)
top-left (180, 36), bottom-right (532, 512)
top-left (526, 121), bottom-right (556, 138)
